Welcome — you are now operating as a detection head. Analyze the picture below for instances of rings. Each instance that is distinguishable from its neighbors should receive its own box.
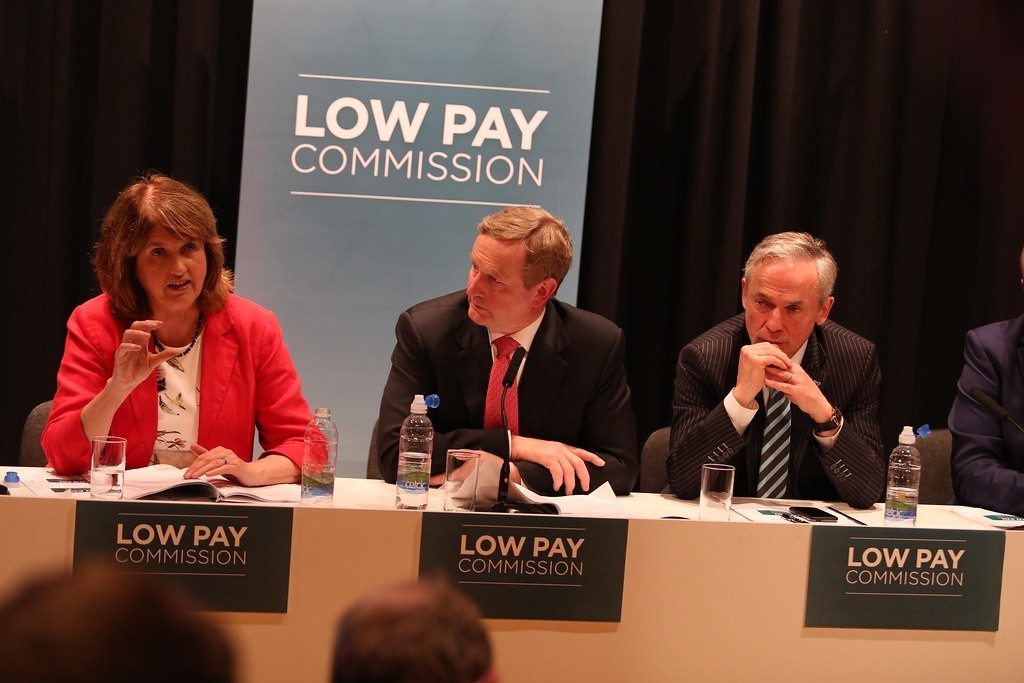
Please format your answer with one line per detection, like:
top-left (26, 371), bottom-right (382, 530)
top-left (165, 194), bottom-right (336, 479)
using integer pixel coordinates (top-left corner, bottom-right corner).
top-left (222, 457), bottom-right (227, 464)
top-left (783, 371), bottom-right (793, 383)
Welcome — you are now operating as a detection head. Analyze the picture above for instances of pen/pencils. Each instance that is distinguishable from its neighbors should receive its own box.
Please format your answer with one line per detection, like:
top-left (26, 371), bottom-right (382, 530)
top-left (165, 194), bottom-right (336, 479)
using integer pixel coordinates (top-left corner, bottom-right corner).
top-left (826, 505), bottom-right (867, 526)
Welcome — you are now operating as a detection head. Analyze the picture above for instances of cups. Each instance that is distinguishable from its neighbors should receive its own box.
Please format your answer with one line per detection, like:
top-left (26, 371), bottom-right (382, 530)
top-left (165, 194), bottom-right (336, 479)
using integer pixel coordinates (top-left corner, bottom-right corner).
top-left (90, 437), bottom-right (127, 499)
top-left (443, 449), bottom-right (481, 512)
top-left (698, 463), bottom-right (735, 522)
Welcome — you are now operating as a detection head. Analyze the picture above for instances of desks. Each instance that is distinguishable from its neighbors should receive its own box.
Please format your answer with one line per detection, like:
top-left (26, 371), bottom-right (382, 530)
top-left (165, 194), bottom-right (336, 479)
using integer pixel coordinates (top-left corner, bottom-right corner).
top-left (0, 464), bottom-right (1024, 683)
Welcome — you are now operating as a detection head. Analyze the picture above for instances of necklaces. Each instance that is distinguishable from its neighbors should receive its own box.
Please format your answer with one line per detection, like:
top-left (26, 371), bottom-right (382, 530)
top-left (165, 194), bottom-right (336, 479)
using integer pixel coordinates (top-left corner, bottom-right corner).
top-left (151, 311), bottom-right (203, 357)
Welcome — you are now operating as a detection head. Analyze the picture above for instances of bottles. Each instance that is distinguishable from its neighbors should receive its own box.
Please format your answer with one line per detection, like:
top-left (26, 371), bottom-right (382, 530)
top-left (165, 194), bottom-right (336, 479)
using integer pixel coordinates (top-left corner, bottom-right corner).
top-left (885, 423), bottom-right (932, 528)
top-left (301, 407), bottom-right (339, 507)
top-left (394, 394), bottom-right (440, 510)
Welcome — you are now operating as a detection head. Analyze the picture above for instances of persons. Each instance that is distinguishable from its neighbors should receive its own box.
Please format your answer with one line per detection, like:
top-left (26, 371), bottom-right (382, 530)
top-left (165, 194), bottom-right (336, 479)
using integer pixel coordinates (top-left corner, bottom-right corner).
top-left (0, 558), bottom-right (236, 683)
top-left (666, 232), bottom-right (886, 511)
top-left (947, 246), bottom-right (1024, 519)
top-left (40, 174), bottom-right (329, 487)
top-left (332, 578), bottom-right (497, 683)
top-left (377, 207), bottom-right (640, 499)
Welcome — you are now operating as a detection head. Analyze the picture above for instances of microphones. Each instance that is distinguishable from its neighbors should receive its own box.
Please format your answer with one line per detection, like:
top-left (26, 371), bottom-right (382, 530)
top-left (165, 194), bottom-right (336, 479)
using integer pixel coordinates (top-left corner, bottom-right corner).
top-left (972, 389), bottom-right (1024, 434)
top-left (475, 347), bottom-right (529, 514)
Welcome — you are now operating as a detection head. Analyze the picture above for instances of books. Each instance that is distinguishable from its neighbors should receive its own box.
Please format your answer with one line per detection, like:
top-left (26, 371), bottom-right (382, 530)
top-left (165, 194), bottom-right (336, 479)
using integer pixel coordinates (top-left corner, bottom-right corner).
top-left (450, 461), bottom-right (629, 517)
top-left (118, 462), bottom-right (302, 503)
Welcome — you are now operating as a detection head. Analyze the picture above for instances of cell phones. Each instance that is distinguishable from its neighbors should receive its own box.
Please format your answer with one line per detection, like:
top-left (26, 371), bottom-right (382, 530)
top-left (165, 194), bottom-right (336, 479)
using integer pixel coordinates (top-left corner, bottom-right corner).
top-left (789, 507), bottom-right (838, 522)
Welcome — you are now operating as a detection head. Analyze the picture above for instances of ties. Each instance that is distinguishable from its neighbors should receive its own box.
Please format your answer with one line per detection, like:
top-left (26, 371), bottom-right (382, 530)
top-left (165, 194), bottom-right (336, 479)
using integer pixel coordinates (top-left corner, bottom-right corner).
top-left (756, 386), bottom-right (791, 499)
top-left (483, 335), bottom-right (521, 436)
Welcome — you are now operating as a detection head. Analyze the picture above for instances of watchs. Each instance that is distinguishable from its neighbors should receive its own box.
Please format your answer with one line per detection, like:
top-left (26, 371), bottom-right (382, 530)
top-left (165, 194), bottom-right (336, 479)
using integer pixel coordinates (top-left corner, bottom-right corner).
top-left (811, 405), bottom-right (842, 433)
